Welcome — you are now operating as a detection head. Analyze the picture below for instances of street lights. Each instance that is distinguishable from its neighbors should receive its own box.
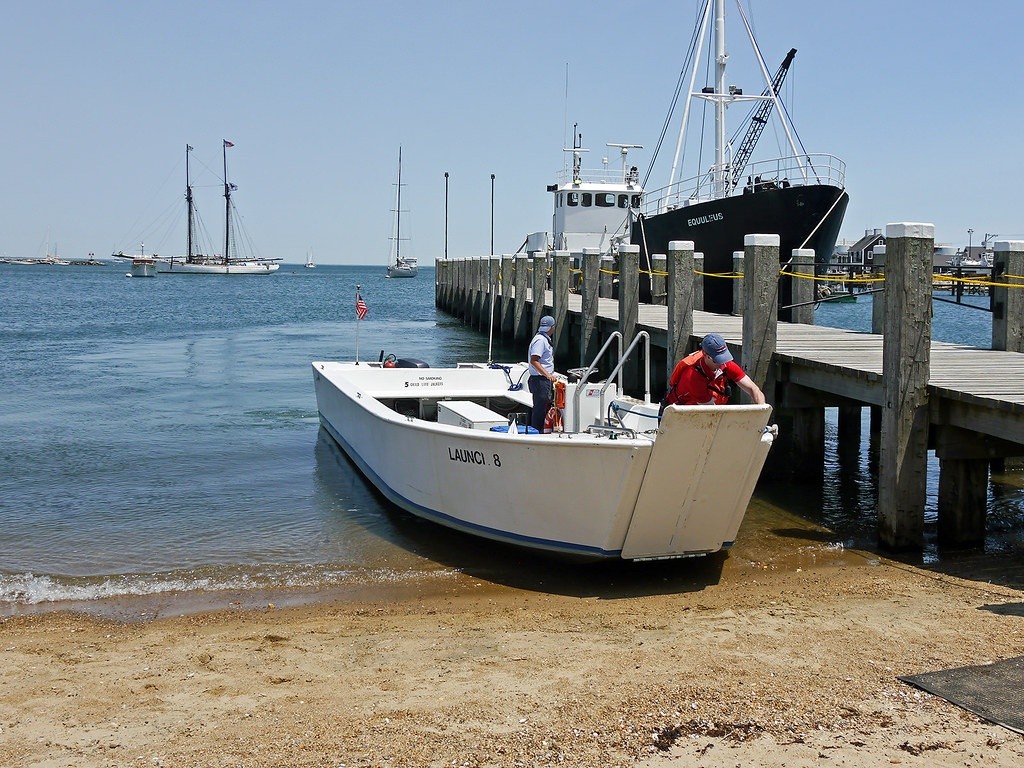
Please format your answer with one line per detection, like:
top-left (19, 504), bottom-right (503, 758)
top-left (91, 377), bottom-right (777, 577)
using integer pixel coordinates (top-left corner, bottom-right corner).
top-left (444, 171), bottom-right (449, 260)
top-left (490, 173), bottom-right (495, 256)
top-left (967, 228), bottom-right (974, 257)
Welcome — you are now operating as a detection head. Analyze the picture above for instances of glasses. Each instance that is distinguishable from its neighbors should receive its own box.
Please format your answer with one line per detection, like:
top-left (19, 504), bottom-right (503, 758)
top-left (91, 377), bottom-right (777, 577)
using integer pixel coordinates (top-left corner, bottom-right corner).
top-left (706, 354), bottom-right (716, 363)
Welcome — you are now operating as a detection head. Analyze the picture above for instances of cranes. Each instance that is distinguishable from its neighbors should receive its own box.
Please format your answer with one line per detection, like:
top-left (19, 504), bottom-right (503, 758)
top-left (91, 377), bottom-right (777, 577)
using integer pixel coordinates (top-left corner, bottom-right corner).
top-left (687, 47), bottom-right (798, 198)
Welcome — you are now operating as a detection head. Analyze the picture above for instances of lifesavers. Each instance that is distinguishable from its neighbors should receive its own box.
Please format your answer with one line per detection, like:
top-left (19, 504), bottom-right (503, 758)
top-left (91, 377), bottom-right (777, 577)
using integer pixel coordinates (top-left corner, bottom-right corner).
top-left (816, 285), bottom-right (832, 299)
top-left (543, 406), bottom-right (565, 435)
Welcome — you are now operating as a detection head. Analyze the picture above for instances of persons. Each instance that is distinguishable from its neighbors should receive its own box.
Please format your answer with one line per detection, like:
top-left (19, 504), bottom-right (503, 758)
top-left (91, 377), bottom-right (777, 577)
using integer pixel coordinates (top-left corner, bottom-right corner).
top-left (658, 333), bottom-right (765, 431)
top-left (528, 316), bottom-right (557, 432)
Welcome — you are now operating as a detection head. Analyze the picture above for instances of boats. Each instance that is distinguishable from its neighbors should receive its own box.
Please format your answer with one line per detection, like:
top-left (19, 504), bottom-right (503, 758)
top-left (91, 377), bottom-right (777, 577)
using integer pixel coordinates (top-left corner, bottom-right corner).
top-left (310, 282), bottom-right (780, 565)
top-left (129, 240), bottom-right (158, 278)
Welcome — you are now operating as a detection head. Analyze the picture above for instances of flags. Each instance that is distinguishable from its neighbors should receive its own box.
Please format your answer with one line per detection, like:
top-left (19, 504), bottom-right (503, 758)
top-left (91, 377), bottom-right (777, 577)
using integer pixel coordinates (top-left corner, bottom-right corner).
top-left (188, 145), bottom-right (194, 151)
top-left (223, 139), bottom-right (234, 147)
top-left (355, 291), bottom-right (368, 321)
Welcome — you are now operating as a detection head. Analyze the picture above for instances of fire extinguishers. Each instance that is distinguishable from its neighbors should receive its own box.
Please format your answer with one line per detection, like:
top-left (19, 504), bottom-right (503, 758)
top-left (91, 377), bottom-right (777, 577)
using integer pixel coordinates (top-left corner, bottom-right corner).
top-left (554, 381), bottom-right (565, 409)
top-left (383, 354), bottom-right (396, 368)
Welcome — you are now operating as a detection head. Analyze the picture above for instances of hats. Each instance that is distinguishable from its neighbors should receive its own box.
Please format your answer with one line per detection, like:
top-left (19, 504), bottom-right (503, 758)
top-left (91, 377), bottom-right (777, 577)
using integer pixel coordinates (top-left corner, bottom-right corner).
top-left (538, 315), bottom-right (555, 332)
top-left (701, 334), bottom-right (734, 365)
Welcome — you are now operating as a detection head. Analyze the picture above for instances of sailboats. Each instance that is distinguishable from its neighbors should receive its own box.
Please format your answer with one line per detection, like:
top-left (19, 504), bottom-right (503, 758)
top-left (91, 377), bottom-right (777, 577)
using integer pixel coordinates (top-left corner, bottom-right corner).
top-left (385, 142), bottom-right (418, 279)
top-left (111, 135), bottom-right (284, 275)
top-left (497, 0), bottom-right (851, 322)
top-left (0, 225), bottom-right (70, 266)
